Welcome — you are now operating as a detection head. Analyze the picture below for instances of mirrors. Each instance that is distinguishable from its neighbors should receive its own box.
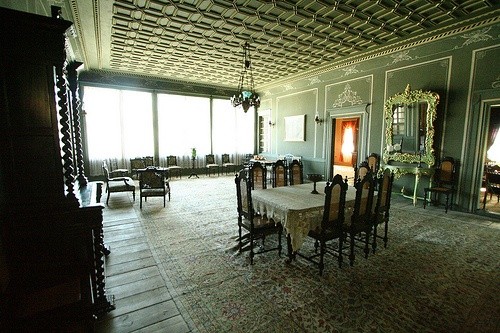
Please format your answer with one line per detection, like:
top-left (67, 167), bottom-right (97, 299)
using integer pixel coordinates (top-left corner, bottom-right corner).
top-left (384, 83), bottom-right (441, 165)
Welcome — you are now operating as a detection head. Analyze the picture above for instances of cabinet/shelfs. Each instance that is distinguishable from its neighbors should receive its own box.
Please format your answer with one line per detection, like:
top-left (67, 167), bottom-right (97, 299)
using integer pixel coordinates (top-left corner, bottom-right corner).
top-left (0, 9), bottom-right (113, 332)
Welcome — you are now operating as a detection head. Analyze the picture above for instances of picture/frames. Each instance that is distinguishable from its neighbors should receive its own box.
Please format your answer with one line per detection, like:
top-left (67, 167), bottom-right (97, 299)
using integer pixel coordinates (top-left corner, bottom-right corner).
top-left (283, 114), bottom-right (306, 142)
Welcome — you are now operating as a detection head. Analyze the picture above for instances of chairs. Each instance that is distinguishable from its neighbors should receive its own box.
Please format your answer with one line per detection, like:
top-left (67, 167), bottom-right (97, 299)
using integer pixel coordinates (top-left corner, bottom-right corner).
top-left (348, 174), bottom-right (371, 260)
top-left (289, 159), bottom-right (303, 185)
top-left (234, 175), bottom-right (283, 265)
top-left (366, 152), bottom-right (380, 169)
top-left (272, 160), bottom-right (288, 187)
top-left (138, 169), bottom-right (170, 208)
top-left (424, 156), bottom-right (458, 214)
top-left (102, 161), bottom-right (136, 205)
top-left (130, 153), bottom-right (253, 177)
top-left (356, 161), bottom-right (371, 189)
top-left (248, 161), bottom-right (267, 190)
top-left (373, 168), bottom-right (394, 253)
top-left (483, 171), bottom-right (500, 209)
top-left (294, 173), bottom-right (348, 275)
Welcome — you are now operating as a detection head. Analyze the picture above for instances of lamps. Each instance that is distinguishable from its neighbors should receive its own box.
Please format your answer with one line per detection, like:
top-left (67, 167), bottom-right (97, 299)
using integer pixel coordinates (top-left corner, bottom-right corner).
top-left (268, 121), bottom-right (275, 128)
top-left (229, 41), bottom-right (260, 113)
top-left (314, 114), bottom-right (321, 124)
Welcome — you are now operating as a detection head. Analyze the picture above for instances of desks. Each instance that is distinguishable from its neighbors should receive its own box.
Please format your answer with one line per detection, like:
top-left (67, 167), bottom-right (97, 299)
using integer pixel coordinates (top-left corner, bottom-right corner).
top-left (379, 164), bottom-right (439, 207)
top-left (249, 180), bottom-right (380, 264)
